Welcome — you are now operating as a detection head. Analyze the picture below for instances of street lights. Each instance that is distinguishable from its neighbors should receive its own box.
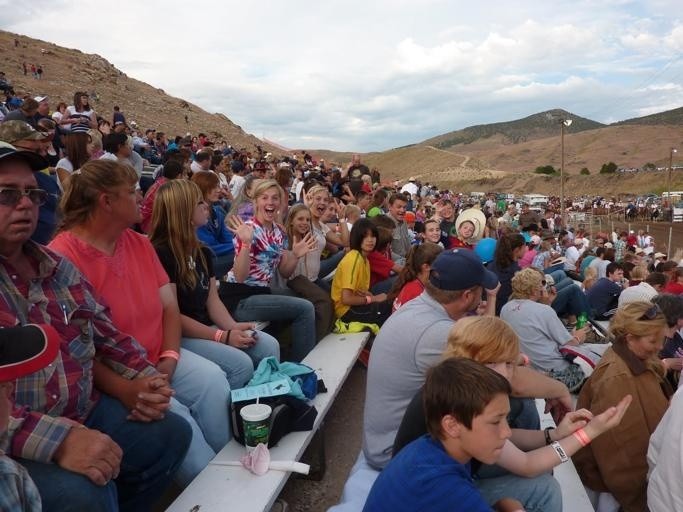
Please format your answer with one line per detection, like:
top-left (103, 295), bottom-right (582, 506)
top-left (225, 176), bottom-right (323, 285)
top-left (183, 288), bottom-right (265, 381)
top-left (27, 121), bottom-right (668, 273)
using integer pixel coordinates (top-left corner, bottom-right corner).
top-left (666, 146), bottom-right (677, 205)
top-left (559, 118), bottom-right (573, 229)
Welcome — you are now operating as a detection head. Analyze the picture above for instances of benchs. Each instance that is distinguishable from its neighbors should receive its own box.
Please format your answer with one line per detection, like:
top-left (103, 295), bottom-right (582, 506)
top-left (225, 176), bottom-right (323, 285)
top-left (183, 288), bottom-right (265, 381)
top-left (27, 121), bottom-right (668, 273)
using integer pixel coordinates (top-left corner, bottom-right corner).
top-left (532, 393), bottom-right (596, 511)
top-left (155, 319), bottom-right (372, 512)
top-left (591, 317), bottom-right (615, 342)
top-left (325, 444), bottom-right (381, 511)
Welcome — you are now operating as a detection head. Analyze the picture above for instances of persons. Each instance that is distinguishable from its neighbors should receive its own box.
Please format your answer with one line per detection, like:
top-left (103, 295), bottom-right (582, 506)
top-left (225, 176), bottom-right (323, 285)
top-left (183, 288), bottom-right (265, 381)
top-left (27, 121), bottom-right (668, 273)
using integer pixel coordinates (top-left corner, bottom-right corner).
top-left (22, 62), bottom-right (43, 80)
top-left (1, 71), bottom-right (683, 512)
top-left (41, 48), bottom-right (45, 56)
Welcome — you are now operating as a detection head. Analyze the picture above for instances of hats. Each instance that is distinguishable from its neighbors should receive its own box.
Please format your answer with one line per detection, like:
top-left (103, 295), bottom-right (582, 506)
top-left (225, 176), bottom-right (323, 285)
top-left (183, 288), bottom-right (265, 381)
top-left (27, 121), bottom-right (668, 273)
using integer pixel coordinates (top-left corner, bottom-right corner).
top-left (542, 231), bottom-right (556, 239)
top-left (0, 140), bottom-right (50, 171)
top-left (0, 120), bottom-right (50, 143)
top-left (655, 252), bottom-right (666, 259)
top-left (33, 96), bottom-right (49, 102)
top-left (429, 247), bottom-right (499, 291)
top-left (146, 128), bottom-right (155, 134)
top-left (603, 242), bottom-right (613, 248)
top-left (455, 208), bottom-right (487, 244)
top-left (530, 235), bottom-right (541, 246)
top-left (1, 323), bottom-right (62, 380)
top-left (636, 247), bottom-right (644, 254)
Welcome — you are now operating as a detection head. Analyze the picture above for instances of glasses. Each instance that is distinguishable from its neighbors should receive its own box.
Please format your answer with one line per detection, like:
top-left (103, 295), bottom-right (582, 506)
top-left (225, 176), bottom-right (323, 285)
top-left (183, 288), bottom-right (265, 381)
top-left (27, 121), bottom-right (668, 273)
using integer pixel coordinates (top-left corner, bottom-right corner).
top-left (12, 143), bottom-right (52, 153)
top-left (639, 302), bottom-right (660, 321)
top-left (541, 279), bottom-right (547, 286)
top-left (0, 186), bottom-right (51, 209)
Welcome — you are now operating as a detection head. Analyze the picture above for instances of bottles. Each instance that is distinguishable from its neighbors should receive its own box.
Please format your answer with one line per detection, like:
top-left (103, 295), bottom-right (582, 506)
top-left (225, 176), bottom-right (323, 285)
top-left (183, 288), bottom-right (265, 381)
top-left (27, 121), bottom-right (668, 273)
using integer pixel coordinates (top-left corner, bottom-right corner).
top-left (576, 311), bottom-right (589, 330)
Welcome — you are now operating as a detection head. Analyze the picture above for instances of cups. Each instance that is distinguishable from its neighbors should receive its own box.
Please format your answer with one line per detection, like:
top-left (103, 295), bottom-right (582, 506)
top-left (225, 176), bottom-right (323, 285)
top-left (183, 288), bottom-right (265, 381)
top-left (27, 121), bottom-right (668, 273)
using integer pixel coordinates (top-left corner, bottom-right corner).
top-left (239, 403), bottom-right (272, 454)
